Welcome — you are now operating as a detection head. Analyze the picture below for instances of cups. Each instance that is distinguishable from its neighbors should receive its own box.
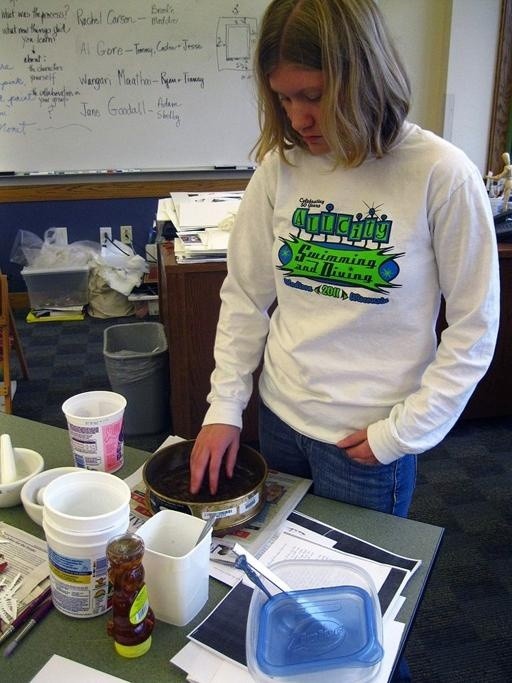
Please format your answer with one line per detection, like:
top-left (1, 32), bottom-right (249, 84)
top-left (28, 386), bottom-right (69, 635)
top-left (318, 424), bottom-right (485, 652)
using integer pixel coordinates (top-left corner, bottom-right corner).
top-left (42, 471), bottom-right (133, 619)
top-left (63, 390), bottom-right (127, 472)
top-left (133, 508), bottom-right (215, 627)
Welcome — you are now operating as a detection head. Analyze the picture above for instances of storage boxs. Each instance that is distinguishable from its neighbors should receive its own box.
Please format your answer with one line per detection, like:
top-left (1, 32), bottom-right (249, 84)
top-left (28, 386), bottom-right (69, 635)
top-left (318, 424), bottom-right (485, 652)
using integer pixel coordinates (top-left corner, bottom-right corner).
top-left (21, 262), bottom-right (91, 310)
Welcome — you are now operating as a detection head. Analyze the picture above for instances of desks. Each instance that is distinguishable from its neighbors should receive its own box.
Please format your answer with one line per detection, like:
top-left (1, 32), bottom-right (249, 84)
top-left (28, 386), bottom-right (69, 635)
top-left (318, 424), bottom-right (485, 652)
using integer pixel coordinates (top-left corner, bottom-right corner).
top-left (156, 239), bottom-right (512, 444)
top-left (0, 412), bottom-right (446, 683)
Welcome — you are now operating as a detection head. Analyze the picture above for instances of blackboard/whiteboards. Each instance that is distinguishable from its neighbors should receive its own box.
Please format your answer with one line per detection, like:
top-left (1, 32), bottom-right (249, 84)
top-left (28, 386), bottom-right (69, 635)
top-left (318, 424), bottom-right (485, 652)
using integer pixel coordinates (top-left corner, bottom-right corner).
top-left (0, 0), bottom-right (452, 177)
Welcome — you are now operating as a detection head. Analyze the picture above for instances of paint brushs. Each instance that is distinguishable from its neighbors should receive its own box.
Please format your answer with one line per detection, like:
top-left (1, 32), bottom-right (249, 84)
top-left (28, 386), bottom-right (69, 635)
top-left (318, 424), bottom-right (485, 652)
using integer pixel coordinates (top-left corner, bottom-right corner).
top-left (1, 586), bottom-right (54, 658)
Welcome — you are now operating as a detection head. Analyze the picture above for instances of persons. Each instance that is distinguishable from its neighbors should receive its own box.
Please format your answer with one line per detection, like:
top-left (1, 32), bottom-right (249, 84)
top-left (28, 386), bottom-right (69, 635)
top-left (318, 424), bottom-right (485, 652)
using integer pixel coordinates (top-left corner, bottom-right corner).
top-left (189, 0), bottom-right (501, 519)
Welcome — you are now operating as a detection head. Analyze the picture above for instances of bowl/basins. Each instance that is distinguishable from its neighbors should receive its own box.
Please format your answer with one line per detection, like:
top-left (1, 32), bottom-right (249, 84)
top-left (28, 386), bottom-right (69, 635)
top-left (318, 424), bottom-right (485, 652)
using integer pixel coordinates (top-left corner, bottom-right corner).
top-left (20, 465), bottom-right (89, 528)
top-left (0, 445), bottom-right (45, 507)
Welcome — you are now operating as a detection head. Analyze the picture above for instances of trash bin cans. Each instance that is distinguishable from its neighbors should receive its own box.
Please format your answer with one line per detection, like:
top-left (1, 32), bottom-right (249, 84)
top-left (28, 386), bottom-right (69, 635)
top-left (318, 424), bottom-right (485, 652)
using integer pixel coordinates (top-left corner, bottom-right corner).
top-left (103, 322), bottom-right (167, 435)
